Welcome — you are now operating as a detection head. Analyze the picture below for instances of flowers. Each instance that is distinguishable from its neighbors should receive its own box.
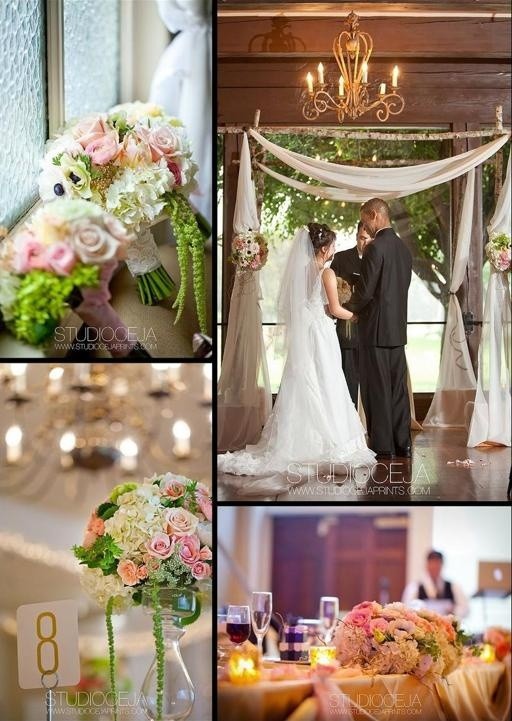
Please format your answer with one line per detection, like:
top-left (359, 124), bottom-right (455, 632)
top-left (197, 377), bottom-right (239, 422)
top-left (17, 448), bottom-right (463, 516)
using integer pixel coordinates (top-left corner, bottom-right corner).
top-left (2, 199), bottom-right (152, 363)
top-left (40, 101), bottom-right (208, 334)
top-left (225, 231), bottom-right (267, 276)
top-left (484, 233), bottom-right (511, 272)
top-left (336, 277), bottom-right (353, 340)
top-left (72, 471), bottom-right (212, 721)
top-left (333, 601), bottom-right (482, 687)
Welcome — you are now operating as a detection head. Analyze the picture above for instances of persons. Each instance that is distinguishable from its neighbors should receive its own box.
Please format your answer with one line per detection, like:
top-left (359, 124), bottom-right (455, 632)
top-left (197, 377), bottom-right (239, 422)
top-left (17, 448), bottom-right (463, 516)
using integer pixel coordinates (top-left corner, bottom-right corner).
top-left (401, 550), bottom-right (469, 621)
top-left (340, 197), bottom-right (414, 460)
top-left (329, 221), bottom-right (375, 412)
top-left (218, 222), bottom-right (378, 477)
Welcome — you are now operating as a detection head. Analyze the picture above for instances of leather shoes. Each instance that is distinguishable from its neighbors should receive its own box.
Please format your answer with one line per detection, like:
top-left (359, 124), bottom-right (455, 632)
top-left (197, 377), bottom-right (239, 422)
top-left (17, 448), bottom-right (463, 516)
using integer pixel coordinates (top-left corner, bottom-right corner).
top-left (393, 446), bottom-right (413, 459)
top-left (372, 448), bottom-right (398, 460)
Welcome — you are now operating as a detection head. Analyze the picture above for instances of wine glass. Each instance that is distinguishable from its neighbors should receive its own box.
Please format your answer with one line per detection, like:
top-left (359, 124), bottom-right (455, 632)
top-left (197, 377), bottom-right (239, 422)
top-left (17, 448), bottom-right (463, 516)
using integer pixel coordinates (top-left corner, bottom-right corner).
top-left (250, 592), bottom-right (274, 675)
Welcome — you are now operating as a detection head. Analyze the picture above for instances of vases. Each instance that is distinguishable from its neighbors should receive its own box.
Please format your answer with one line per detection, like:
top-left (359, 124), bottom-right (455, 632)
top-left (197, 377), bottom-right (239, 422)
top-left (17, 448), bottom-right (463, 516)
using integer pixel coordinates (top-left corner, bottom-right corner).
top-left (137, 588), bottom-right (196, 721)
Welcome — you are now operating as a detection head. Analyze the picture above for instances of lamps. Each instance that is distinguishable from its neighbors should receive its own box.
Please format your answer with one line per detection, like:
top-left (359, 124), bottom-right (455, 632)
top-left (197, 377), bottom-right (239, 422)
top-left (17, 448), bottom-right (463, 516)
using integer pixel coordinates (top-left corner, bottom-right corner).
top-left (301, 11), bottom-right (405, 122)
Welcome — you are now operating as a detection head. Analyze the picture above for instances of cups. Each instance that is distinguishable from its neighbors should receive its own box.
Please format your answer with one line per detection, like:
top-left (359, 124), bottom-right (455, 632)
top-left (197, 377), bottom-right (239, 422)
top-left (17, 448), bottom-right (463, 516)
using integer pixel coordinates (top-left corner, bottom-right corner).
top-left (226, 607), bottom-right (251, 648)
top-left (280, 596), bottom-right (339, 674)
